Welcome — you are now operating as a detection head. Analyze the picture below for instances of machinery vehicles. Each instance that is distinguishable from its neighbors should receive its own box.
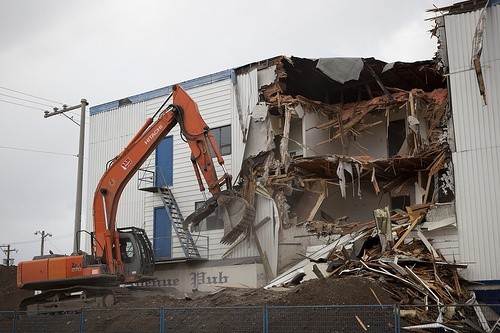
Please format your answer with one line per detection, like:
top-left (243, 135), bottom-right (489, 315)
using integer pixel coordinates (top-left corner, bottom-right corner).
top-left (12, 83), bottom-right (257, 316)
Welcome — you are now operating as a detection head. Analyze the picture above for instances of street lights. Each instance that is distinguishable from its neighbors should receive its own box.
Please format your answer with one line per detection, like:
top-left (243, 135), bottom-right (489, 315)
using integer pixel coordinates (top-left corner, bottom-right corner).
top-left (33, 230), bottom-right (53, 257)
